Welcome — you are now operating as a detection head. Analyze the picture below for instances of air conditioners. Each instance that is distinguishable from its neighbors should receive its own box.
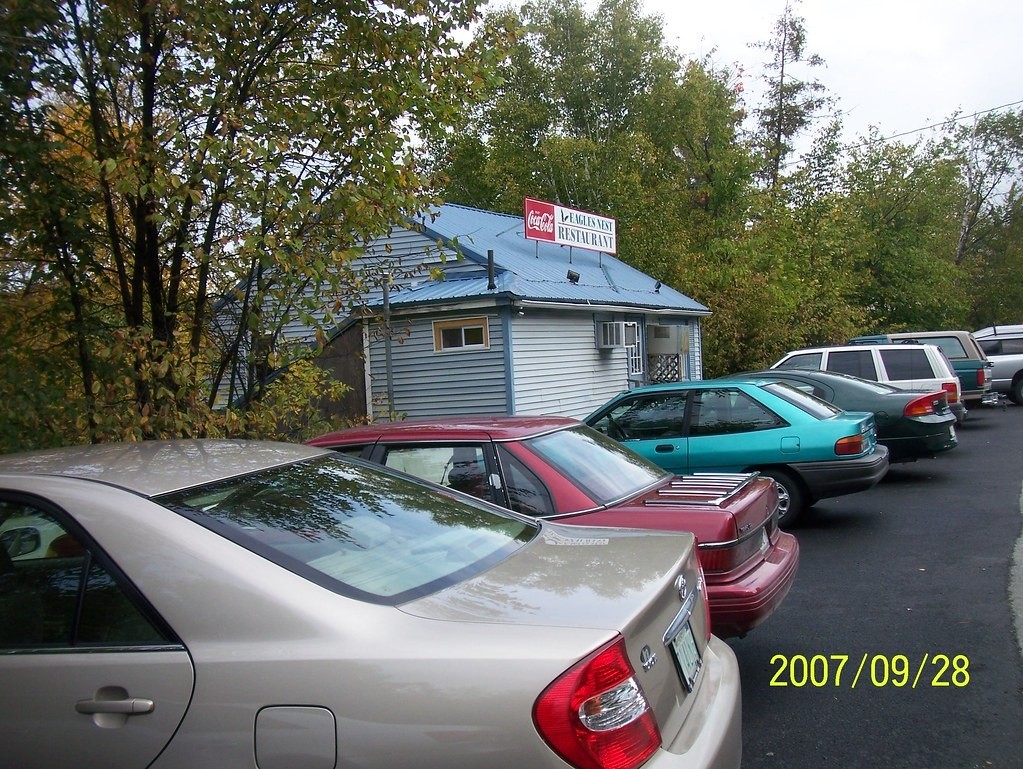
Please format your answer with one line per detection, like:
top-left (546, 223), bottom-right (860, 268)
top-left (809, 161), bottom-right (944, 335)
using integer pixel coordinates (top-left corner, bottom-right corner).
top-left (596, 321), bottom-right (637, 348)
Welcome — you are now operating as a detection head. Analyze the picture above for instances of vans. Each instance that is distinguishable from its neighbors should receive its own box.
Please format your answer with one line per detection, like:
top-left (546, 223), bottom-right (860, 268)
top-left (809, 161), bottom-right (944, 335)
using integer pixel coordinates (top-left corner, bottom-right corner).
top-left (770, 344), bottom-right (971, 425)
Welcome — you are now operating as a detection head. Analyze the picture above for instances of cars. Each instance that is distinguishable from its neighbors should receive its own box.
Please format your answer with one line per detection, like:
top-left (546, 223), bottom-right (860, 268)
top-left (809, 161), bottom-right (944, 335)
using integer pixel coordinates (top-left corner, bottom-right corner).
top-left (708, 369), bottom-right (958, 464)
top-left (221, 417), bottom-right (801, 638)
top-left (0, 437), bottom-right (743, 769)
top-left (578, 378), bottom-right (890, 528)
top-left (965, 325), bottom-right (1023, 407)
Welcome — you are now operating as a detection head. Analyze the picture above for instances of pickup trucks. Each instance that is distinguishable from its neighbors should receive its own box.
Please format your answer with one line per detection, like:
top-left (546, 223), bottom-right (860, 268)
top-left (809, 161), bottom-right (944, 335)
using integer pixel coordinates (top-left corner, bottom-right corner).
top-left (849, 332), bottom-right (1000, 405)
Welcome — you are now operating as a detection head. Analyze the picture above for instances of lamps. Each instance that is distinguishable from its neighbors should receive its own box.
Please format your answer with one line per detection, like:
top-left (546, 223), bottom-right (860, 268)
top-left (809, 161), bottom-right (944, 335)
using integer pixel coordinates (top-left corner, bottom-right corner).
top-left (567, 269), bottom-right (580, 285)
top-left (654, 281), bottom-right (661, 292)
